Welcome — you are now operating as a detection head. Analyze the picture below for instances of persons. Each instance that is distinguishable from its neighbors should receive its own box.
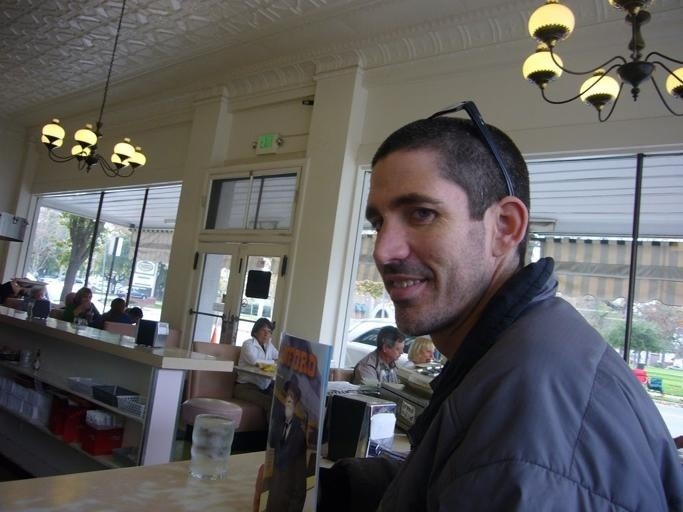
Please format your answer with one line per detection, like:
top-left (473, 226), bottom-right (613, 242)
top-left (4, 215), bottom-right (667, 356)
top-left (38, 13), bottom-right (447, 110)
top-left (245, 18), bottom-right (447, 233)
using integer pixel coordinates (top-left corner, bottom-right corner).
top-left (631, 362), bottom-right (649, 393)
top-left (230, 317), bottom-right (279, 427)
top-left (0, 277), bottom-right (142, 330)
top-left (265, 374), bottom-right (307, 512)
top-left (351, 325), bottom-right (406, 386)
top-left (315, 99), bottom-right (682, 511)
top-left (403, 336), bottom-right (437, 370)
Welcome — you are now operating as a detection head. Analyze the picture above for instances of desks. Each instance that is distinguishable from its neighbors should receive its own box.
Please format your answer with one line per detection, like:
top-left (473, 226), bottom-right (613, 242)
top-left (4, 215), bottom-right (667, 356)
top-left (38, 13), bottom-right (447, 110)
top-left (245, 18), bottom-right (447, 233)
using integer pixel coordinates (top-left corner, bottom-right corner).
top-left (0, 433), bottom-right (410, 512)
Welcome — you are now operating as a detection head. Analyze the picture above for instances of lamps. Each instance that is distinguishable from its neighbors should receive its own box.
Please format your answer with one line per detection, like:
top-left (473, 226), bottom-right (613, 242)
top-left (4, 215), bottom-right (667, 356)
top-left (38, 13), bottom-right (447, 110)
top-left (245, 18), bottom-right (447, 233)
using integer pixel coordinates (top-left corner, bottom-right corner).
top-left (522, 0), bottom-right (682, 124)
top-left (39, 0), bottom-right (146, 178)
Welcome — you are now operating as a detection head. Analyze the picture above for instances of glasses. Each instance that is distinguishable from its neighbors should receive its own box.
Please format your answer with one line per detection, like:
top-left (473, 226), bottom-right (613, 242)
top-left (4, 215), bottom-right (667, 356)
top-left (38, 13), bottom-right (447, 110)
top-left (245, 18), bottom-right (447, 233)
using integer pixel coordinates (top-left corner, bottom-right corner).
top-left (428, 98), bottom-right (515, 196)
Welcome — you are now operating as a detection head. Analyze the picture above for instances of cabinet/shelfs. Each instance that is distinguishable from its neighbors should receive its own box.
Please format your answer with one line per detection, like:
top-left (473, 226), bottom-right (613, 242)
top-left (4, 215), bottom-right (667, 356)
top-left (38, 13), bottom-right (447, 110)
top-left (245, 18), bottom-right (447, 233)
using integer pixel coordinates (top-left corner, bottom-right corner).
top-left (0, 305), bottom-right (233, 479)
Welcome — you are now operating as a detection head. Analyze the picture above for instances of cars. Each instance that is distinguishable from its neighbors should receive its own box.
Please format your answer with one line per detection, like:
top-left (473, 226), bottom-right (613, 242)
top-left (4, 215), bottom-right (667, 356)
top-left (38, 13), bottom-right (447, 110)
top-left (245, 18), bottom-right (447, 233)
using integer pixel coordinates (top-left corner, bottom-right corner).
top-left (341, 315), bottom-right (447, 376)
top-left (58, 270), bottom-right (149, 301)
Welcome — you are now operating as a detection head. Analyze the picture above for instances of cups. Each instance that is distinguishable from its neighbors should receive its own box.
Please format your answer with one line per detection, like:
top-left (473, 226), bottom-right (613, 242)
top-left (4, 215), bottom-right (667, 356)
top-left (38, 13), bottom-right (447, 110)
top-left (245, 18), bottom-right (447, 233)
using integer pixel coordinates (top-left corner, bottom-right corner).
top-left (189, 414), bottom-right (235, 481)
top-left (19, 349), bottom-right (33, 368)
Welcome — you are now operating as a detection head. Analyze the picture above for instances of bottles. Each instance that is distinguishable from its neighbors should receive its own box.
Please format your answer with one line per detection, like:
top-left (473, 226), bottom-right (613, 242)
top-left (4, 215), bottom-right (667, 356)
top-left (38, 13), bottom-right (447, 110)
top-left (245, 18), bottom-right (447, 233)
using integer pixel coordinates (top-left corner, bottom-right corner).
top-left (30, 347), bottom-right (42, 376)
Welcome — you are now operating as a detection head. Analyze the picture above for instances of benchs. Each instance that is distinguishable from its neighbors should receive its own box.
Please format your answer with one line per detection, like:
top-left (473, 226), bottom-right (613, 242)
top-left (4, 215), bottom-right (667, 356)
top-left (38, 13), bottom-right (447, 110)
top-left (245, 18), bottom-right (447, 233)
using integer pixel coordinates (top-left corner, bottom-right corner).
top-left (326, 368), bottom-right (354, 406)
top-left (101, 320), bottom-right (268, 444)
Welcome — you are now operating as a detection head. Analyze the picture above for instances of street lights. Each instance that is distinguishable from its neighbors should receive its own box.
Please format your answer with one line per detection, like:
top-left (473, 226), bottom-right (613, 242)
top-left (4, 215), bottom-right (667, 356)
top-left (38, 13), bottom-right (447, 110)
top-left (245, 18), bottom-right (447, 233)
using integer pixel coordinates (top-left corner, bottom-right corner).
top-left (99, 221), bottom-right (133, 314)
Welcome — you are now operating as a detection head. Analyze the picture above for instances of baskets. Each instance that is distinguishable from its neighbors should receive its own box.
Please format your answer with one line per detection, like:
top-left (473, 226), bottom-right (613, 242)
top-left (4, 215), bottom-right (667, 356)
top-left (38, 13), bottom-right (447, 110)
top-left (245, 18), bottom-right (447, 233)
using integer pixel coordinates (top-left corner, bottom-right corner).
top-left (116, 394), bottom-right (149, 418)
top-left (68, 376), bottom-right (115, 398)
top-left (92, 385), bottom-right (140, 408)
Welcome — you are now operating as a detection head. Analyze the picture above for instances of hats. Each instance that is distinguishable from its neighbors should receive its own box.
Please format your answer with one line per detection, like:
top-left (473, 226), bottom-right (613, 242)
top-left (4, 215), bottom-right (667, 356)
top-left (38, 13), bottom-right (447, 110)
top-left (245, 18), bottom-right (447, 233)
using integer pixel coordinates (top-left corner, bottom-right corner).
top-left (284, 374), bottom-right (301, 402)
